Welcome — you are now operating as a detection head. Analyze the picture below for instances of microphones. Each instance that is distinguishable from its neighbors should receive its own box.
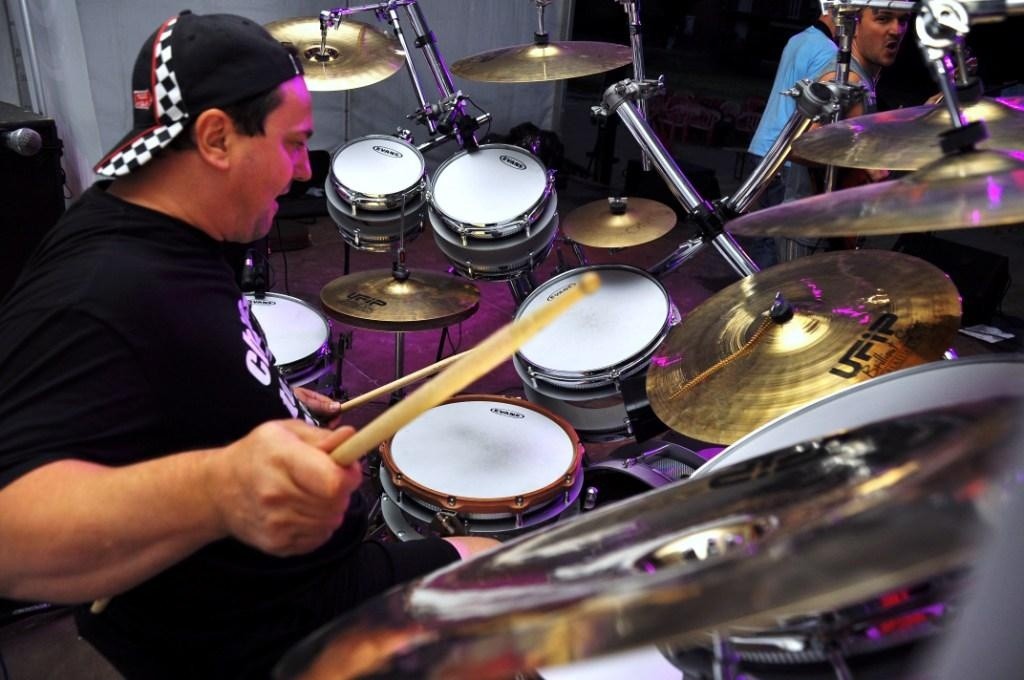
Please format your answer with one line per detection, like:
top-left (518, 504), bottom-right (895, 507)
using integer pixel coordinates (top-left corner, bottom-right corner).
top-left (0, 127), bottom-right (42, 156)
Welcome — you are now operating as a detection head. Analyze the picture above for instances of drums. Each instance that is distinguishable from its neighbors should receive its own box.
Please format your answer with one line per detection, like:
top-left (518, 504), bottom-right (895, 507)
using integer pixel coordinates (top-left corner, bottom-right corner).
top-left (378, 389), bottom-right (587, 555)
top-left (673, 346), bottom-right (1024, 667)
top-left (507, 262), bottom-right (682, 446)
top-left (241, 288), bottom-right (333, 392)
top-left (324, 133), bottom-right (430, 256)
top-left (425, 142), bottom-right (562, 283)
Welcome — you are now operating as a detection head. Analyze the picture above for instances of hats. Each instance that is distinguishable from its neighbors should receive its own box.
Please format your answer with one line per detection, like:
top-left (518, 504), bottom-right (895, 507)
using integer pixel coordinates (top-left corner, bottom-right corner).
top-left (93, 9), bottom-right (304, 179)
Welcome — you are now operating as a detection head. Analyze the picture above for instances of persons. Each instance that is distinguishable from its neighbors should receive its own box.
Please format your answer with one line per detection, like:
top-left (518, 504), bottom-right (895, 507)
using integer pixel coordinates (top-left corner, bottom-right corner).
top-left (0, 6), bottom-right (507, 680)
top-left (740, 0), bottom-right (844, 269)
top-left (781, 0), bottom-right (913, 255)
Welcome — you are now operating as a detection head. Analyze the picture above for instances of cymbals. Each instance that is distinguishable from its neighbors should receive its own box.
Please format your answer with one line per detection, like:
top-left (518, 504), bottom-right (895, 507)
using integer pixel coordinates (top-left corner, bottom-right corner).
top-left (449, 40), bottom-right (633, 83)
top-left (788, 98), bottom-right (1024, 173)
top-left (645, 249), bottom-right (965, 447)
top-left (561, 193), bottom-right (677, 248)
top-left (261, 15), bottom-right (406, 93)
top-left (320, 264), bottom-right (480, 333)
top-left (273, 389), bottom-right (1024, 680)
top-left (722, 151), bottom-right (1023, 238)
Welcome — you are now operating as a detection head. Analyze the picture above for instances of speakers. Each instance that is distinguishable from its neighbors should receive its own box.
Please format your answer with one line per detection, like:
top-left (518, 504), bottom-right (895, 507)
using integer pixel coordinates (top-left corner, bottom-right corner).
top-left (0, 101), bottom-right (67, 255)
